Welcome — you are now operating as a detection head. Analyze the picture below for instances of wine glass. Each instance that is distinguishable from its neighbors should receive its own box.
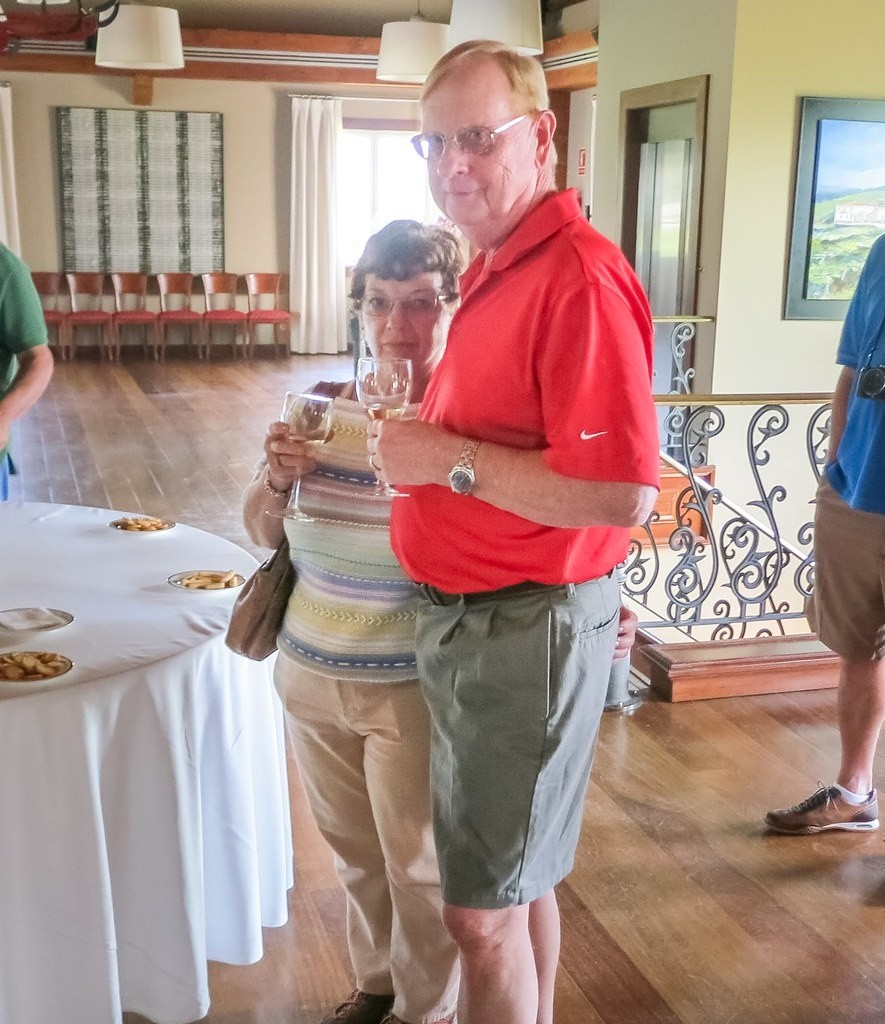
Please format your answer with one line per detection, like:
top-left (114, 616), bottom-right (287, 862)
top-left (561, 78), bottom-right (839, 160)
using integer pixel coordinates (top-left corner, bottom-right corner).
top-left (356, 359), bottom-right (413, 496)
top-left (264, 391), bottom-right (332, 523)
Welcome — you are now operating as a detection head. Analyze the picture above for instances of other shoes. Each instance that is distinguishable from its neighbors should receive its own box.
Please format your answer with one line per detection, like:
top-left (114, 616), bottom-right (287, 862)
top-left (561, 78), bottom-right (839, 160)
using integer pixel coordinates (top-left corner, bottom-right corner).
top-left (319, 985), bottom-right (455, 1024)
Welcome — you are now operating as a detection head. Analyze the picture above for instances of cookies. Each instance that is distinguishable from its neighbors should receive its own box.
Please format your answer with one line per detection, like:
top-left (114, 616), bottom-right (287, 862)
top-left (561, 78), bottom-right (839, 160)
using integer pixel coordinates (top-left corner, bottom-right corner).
top-left (121, 517), bottom-right (164, 531)
top-left (0, 651), bottom-right (64, 680)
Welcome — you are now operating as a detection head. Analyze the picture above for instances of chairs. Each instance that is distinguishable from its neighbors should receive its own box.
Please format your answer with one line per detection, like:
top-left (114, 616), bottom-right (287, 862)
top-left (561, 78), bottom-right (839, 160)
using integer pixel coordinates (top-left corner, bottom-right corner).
top-left (30, 272), bottom-right (66, 360)
top-left (244, 272), bottom-right (291, 359)
top-left (109, 272), bottom-right (158, 360)
top-left (156, 272), bottom-right (203, 361)
top-left (200, 272), bottom-right (247, 360)
top-left (65, 272), bottom-right (113, 361)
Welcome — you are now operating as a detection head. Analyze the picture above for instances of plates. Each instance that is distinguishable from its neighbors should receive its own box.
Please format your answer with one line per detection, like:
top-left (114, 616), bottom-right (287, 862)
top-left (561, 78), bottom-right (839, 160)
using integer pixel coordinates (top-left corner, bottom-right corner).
top-left (0, 651), bottom-right (73, 682)
top-left (168, 570), bottom-right (246, 592)
top-left (0, 608), bottom-right (74, 636)
top-left (105, 518), bottom-right (176, 535)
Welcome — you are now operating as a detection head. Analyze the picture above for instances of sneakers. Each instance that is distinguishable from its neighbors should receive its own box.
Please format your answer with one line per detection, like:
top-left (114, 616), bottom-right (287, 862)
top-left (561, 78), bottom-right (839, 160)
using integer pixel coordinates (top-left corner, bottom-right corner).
top-left (763, 783), bottom-right (881, 833)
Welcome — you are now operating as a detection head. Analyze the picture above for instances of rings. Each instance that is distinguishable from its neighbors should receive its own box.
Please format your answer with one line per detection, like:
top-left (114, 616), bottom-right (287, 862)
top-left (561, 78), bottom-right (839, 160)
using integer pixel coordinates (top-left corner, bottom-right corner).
top-left (267, 423), bottom-right (273, 436)
top-left (276, 454), bottom-right (283, 467)
top-left (368, 455), bottom-right (377, 471)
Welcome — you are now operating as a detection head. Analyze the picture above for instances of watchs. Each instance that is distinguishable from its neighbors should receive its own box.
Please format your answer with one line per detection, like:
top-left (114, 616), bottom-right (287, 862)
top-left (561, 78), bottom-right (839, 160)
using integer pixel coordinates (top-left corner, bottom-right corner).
top-left (446, 440), bottom-right (480, 496)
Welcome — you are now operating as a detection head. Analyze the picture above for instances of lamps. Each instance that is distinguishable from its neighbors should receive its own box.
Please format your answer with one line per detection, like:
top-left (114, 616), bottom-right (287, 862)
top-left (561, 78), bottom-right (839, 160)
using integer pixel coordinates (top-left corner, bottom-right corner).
top-left (445, 0), bottom-right (543, 56)
top-left (95, 5), bottom-right (185, 69)
top-left (0, 0), bottom-right (123, 57)
top-left (375, 0), bottom-right (450, 83)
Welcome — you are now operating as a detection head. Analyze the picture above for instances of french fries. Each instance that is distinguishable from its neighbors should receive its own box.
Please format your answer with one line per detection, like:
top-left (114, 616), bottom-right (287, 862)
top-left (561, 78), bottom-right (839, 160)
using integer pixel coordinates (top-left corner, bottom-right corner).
top-left (180, 569), bottom-right (238, 589)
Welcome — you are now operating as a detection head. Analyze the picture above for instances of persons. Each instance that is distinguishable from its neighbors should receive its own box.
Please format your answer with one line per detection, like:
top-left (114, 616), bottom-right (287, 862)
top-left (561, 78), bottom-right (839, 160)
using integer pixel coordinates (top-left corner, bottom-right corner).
top-left (365, 40), bottom-right (660, 1024)
top-left (0, 241), bottom-right (54, 502)
top-left (243, 219), bottom-right (639, 1024)
top-left (764, 235), bottom-right (885, 835)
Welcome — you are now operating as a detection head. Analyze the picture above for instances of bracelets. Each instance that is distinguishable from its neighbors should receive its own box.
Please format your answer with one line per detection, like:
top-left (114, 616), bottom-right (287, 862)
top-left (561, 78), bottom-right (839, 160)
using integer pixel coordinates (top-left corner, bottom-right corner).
top-left (263, 468), bottom-right (293, 499)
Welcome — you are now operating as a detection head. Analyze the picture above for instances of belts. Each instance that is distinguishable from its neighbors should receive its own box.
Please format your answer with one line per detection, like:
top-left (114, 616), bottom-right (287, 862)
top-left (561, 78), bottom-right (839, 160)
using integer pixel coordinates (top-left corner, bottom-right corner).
top-left (410, 563), bottom-right (614, 606)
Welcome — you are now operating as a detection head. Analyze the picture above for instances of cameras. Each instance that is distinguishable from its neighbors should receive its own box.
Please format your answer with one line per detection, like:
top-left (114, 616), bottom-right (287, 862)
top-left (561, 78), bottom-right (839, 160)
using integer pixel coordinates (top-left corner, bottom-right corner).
top-left (855, 363), bottom-right (885, 401)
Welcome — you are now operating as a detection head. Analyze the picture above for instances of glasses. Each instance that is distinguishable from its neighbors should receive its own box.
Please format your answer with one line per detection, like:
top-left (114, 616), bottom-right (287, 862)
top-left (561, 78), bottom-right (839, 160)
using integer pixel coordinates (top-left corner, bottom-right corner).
top-left (356, 292), bottom-right (451, 318)
top-left (409, 114), bottom-right (528, 161)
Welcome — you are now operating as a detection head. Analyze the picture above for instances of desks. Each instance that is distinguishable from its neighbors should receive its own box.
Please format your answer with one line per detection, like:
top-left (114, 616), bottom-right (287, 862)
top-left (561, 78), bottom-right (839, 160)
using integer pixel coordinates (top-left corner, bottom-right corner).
top-left (0, 501), bottom-right (294, 1024)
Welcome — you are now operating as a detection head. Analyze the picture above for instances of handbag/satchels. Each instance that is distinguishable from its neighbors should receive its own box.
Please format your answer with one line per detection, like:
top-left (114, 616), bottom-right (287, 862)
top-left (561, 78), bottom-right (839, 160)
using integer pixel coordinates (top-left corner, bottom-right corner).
top-left (225, 535), bottom-right (296, 662)
